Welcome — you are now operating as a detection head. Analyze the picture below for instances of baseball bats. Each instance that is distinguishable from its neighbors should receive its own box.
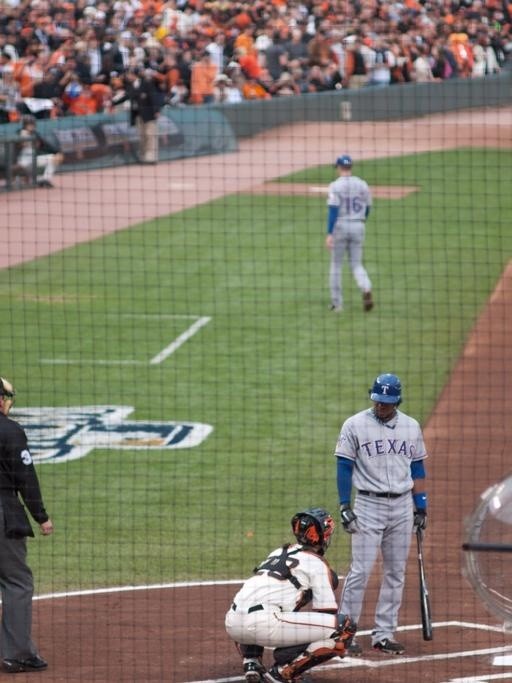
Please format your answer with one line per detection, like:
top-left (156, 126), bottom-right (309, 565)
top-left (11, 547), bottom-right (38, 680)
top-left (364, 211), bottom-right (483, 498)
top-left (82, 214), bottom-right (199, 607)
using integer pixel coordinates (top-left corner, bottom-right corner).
top-left (415, 531), bottom-right (433, 641)
top-left (461, 539), bottom-right (509, 554)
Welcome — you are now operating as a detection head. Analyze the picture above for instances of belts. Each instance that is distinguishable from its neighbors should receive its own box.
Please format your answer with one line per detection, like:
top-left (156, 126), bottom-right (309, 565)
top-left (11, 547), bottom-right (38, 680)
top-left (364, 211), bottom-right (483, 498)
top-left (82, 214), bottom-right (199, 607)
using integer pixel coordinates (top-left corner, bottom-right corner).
top-left (357, 489), bottom-right (402, 500)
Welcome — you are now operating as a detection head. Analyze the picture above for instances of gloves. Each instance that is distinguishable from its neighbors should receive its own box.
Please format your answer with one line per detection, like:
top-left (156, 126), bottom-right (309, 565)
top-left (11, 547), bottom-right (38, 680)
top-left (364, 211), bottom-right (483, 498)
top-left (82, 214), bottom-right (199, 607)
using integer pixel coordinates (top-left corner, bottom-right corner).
top-left (412, 510), bottom-right (429, 534)
top-left (340, 503), bottom-right (361, 535)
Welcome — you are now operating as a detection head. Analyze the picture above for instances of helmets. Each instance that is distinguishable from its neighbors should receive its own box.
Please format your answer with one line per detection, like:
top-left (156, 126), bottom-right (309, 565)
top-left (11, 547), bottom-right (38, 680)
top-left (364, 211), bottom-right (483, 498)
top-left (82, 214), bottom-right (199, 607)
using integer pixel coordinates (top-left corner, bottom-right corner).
top-left (290, 508), bottom-right (336, 547)
top-left (369, 374), bottom-right (402, 405)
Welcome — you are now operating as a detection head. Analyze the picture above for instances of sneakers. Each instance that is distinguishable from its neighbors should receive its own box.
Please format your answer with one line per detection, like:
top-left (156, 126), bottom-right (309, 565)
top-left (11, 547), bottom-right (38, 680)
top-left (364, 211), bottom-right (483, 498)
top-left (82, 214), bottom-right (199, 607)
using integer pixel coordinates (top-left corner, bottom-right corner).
top-left (2, 653), bottom-right (49, 674)
top-left (361, 287), bottom-right (375, 313)
top-left (263, 664), bottom-right (296, 683)
top-left (373, 638), bottom-right (406, 656)
top-left (242, 658), bottom-right (262, 683)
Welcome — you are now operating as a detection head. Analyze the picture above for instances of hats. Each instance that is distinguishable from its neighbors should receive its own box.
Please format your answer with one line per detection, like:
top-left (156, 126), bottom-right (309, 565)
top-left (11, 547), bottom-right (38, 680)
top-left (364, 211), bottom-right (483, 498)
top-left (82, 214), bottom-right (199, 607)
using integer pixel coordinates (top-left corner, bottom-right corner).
top-left (335, 154), bottom-right (352, 169)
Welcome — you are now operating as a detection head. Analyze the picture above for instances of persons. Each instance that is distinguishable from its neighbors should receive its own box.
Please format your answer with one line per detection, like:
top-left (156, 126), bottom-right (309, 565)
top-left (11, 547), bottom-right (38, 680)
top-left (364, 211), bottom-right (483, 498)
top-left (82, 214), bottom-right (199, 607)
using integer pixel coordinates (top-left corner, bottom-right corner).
top-left (326, 155), bottom-right (374, 315)
top-left (109, 65), bottom-right (168, 165)
top-left (334, 374), bottom-right (429, 659)
top-left (0, 378), bottom-right (54, 674)
top-left (226, 506), bottom-right (357, 682)
top-left (10, 114), bottom-right (64, 187)
top-left (0, 0), bottom-right (512, 124)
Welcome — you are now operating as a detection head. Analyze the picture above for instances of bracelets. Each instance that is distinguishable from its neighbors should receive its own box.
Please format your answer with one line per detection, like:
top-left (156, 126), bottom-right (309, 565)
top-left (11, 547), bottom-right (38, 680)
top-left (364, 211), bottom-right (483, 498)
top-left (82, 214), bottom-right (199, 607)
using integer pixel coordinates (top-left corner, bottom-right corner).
top-left (414, 493), bottom-right (427, 509)
